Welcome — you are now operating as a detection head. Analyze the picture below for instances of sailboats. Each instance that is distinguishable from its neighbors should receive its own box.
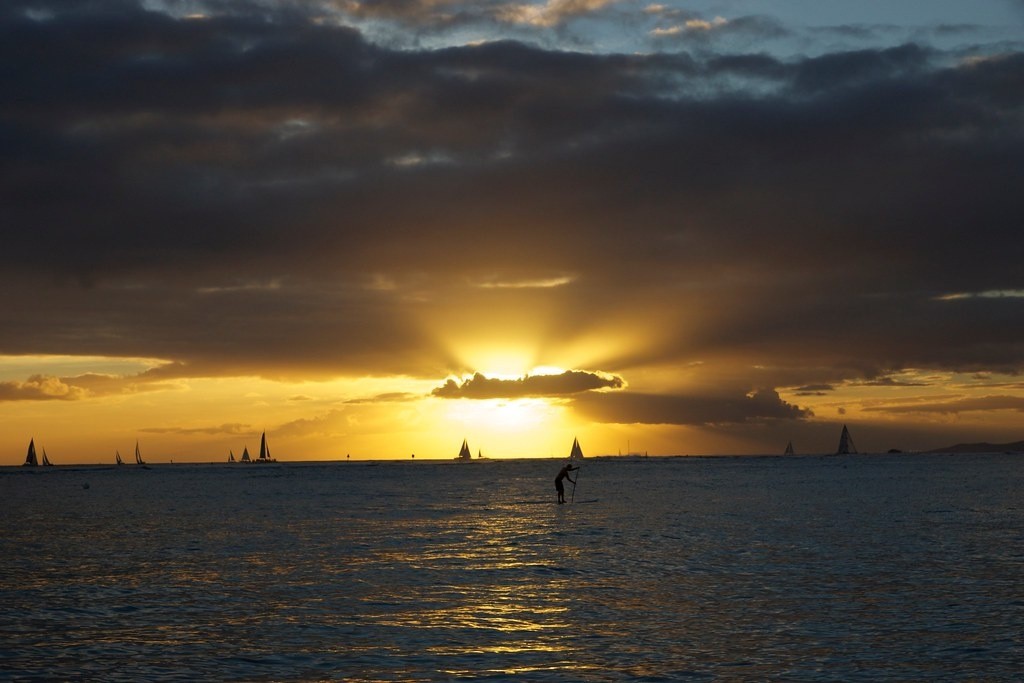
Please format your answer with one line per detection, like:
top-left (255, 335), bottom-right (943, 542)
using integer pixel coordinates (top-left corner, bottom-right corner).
top-left (134, 439), bottom-right (146, 464)
top-left (837, 424), bottom-right (858, 456)
top-left (21, 436), bottom-right (38, 467)
top-left (42, 447), bottom-right (54, 466)
top-left (115, 450), bottom-right (125, 465)
top-left (227, 429), bottom-right (276, 463)
top-left (782, 440), bottom-right (794, 458)
top-left (570, 436), bottom-right (584, 461)
top-left (454, 439), bottom-right (473, 463)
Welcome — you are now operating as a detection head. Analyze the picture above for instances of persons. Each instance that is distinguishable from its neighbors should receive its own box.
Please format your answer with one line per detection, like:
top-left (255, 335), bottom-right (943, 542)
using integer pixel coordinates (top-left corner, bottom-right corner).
top-left (554, 463), bottom-right (580, 501)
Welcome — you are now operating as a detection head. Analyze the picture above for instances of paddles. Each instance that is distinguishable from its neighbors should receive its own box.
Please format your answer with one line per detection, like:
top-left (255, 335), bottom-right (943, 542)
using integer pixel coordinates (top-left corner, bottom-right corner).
top-left (571, 467), bottom-right (580, 503)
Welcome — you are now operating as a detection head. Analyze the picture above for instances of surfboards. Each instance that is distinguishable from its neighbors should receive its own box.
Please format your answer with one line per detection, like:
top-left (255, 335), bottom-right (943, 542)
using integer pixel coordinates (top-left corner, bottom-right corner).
top-left (507, 498), bottom-right (599, 504)
